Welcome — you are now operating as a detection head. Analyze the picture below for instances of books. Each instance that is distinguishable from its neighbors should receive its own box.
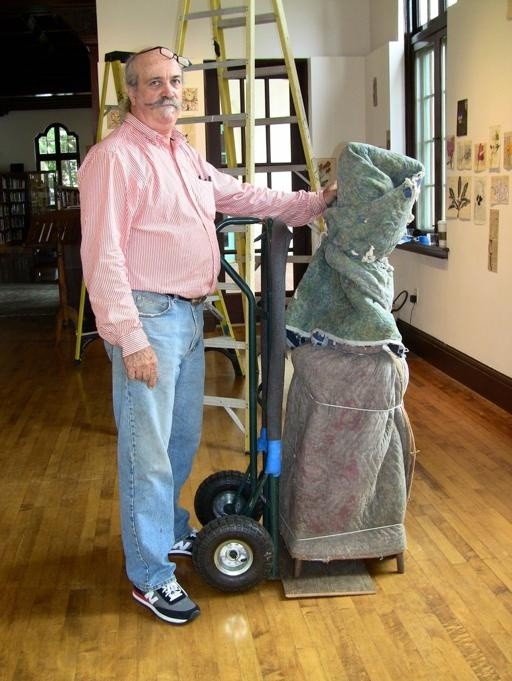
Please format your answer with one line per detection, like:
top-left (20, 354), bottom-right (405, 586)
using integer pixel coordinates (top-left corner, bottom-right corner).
top-left (0, 177), bottom-right (80, 245)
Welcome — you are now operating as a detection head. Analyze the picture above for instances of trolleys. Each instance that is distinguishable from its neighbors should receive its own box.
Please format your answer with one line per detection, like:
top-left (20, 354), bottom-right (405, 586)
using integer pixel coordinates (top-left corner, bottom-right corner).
top-left (190, 218), bottom-right (293, 593)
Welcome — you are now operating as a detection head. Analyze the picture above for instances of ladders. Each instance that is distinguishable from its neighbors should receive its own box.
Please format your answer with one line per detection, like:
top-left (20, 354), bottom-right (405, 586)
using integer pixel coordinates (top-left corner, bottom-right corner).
top-left (76, 47), bottom-right (245, 382)
top-left (174, 1), bottom-right (328, 454)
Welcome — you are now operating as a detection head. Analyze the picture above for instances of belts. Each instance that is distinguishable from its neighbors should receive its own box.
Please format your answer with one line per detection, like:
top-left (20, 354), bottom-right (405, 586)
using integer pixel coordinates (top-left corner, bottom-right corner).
top-left (166, 294), bottom-right (207, 306)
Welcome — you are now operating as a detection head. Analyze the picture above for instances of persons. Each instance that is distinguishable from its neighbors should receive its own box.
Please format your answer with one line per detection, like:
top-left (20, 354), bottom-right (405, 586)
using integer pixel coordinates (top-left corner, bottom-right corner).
top-left (76, 46), bottom-right (338, 624)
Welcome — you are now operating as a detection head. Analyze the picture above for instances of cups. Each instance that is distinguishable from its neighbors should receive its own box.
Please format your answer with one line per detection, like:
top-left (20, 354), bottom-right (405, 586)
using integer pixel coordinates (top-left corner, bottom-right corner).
top-left (438, 220), bottom-right (448, 248)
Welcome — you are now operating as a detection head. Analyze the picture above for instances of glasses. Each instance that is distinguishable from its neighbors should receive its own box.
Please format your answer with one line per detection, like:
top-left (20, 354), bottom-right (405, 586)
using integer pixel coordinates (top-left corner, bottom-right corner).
top-left (135, 46), bottom-right (192, 69)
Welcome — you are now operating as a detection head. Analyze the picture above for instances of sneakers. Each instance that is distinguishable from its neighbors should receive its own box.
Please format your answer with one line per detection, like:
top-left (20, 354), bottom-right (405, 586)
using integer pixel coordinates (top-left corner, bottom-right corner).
top-left (169, 528), bottom-right (198, 556)
top-left (132, 580), bottom-right (201, 625)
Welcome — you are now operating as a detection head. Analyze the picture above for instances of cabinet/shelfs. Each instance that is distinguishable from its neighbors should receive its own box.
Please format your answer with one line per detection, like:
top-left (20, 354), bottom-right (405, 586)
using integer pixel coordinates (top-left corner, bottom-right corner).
top-left (0, 170), bottom-right (33, 248)
